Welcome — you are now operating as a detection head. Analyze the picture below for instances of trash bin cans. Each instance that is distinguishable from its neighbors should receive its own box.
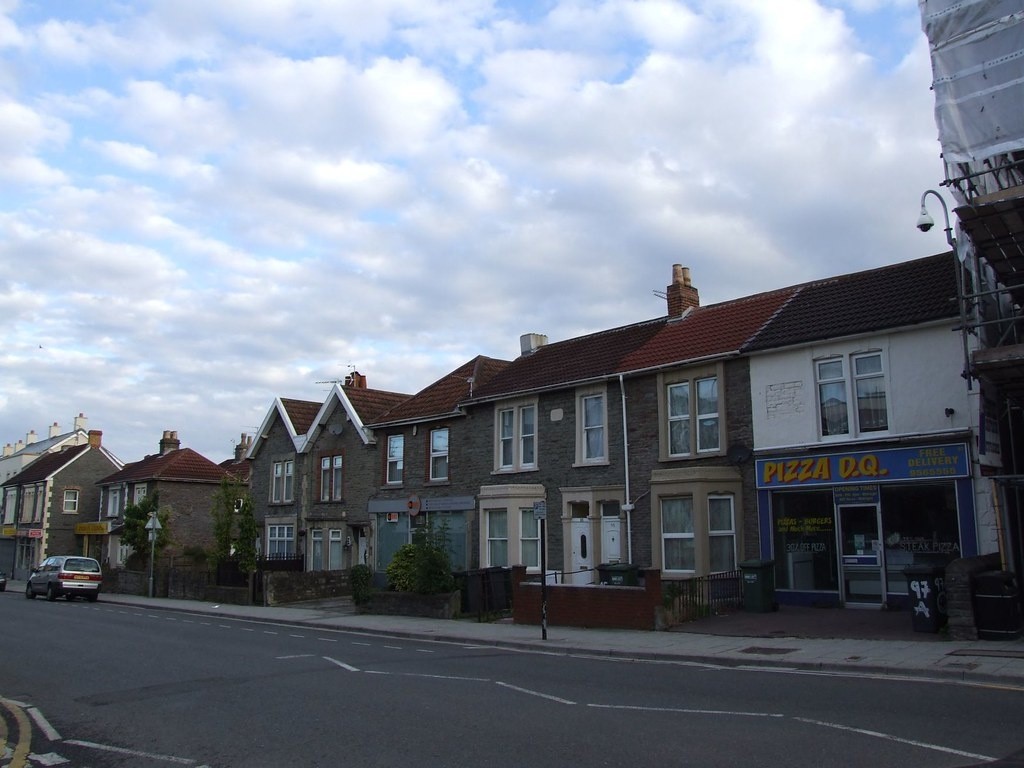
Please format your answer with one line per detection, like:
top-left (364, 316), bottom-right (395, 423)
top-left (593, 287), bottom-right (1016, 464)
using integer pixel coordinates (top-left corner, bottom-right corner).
top-left (903, 564), bottom-right (949, 634)
top-left (595, 561), bottom-right (641, 587)
top-left (976, 571), bottom-right (1023, 640)
top-left (740, 559), bottom-right (780, 614)
top-left (451, 570), bottom-right (485, 606)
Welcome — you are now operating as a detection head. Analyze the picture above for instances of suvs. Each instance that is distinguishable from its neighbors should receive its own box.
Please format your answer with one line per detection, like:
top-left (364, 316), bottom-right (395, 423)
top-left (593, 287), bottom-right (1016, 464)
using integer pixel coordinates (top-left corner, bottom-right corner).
top-left (26, 555), bottom-right (103, 603)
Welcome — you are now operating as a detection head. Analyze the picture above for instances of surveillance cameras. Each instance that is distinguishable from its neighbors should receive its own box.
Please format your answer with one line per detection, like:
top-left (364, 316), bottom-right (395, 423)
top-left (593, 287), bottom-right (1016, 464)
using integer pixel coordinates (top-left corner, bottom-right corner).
top-left (916, 214), bottom-right (934, 233)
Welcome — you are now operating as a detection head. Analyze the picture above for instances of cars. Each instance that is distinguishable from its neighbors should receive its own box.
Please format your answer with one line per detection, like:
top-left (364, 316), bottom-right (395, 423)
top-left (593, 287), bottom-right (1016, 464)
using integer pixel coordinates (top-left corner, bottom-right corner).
top-left (0, 570), bottom-right (6, 591)
